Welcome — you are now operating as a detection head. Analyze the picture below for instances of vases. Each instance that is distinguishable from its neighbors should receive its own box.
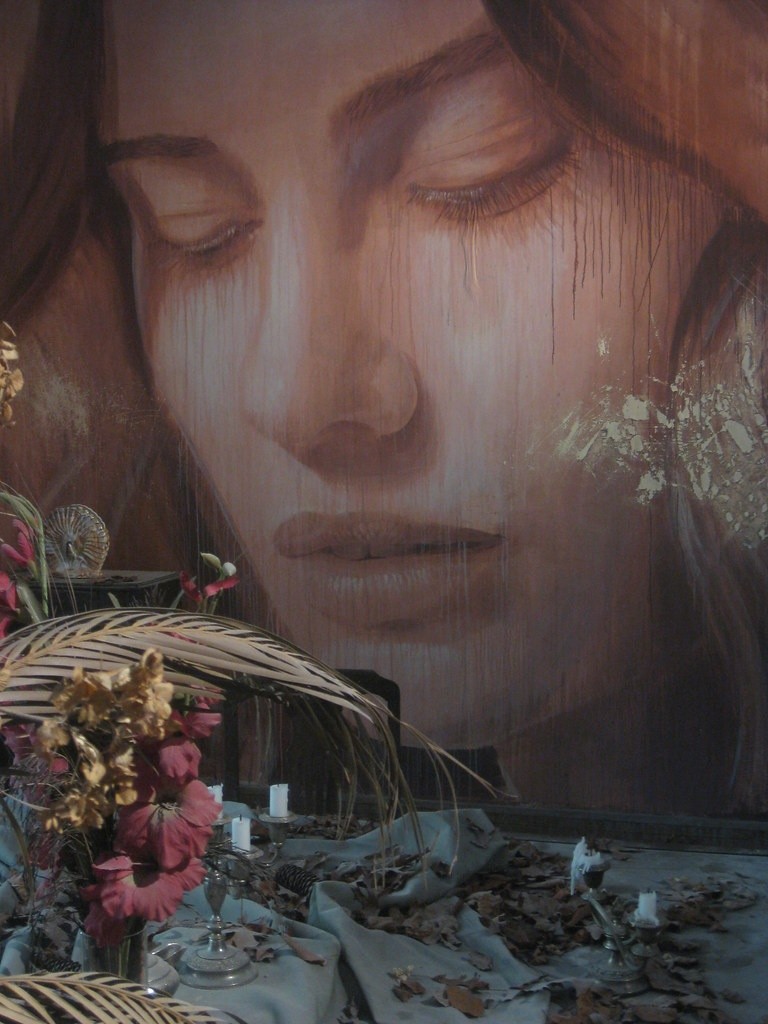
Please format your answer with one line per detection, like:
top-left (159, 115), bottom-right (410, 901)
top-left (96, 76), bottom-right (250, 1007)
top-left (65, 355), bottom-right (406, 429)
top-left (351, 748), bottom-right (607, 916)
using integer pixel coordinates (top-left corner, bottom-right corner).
top-left (80, 929), bottom-right (150, 987)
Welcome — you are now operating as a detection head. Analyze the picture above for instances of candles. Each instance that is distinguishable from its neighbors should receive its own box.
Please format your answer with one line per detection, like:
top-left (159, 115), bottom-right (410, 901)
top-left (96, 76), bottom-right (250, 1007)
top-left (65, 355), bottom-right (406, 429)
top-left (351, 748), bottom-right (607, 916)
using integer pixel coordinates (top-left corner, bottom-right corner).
top-left (570, 836), bottom-right (600, 895)
top-left (231, 814), bottom-right (251, 855)
top-left (634, 888), bottom-right (659, 926)
top-left (270, 783), bottom-right (289, 818)
top-left (205, 782), bottom-right (224, 821)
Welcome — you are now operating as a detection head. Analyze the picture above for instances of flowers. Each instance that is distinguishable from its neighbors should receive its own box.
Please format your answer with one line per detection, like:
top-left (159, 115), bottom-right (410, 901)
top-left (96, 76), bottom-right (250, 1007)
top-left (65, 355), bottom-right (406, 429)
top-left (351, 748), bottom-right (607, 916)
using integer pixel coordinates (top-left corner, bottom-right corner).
top-left (0, 481), bottom-right (238, 978)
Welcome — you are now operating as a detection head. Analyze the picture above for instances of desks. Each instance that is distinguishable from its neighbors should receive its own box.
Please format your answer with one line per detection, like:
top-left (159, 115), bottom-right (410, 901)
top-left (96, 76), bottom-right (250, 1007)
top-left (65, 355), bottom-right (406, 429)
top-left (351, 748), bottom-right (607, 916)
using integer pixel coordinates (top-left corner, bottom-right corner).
top-left (0, 810), bottom-right (767, 1024)
top-left (26, 569), bottom-right (183, 616)
